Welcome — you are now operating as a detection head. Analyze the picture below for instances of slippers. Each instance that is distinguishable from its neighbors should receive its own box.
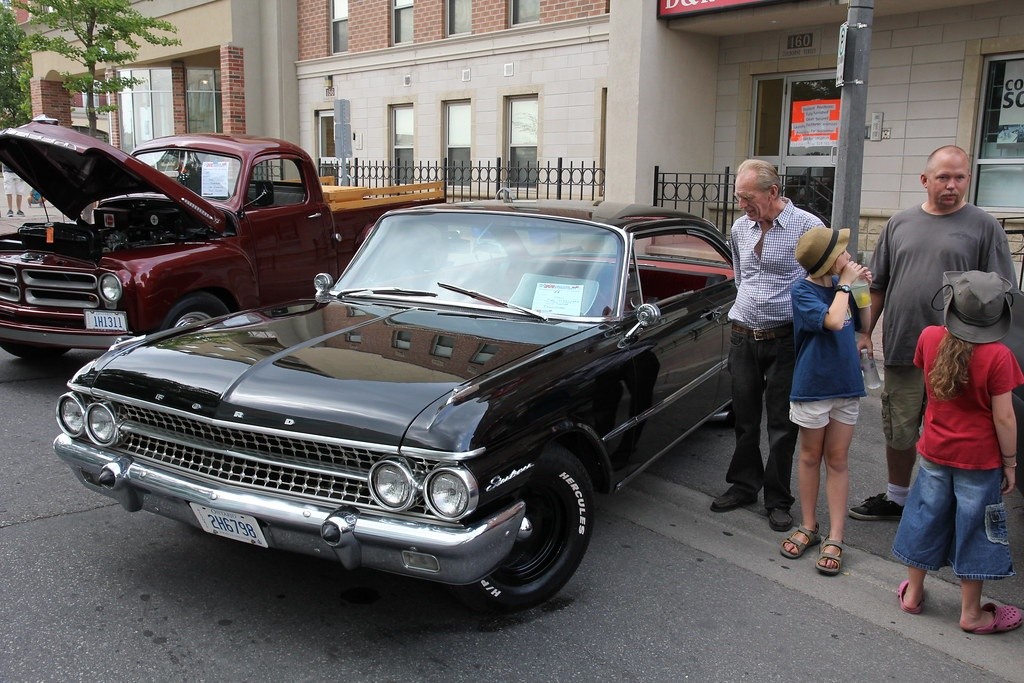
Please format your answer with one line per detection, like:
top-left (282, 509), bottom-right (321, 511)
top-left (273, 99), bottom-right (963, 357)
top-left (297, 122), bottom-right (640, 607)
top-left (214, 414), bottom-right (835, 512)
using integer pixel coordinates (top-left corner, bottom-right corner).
top-left (898, 579), bottom-right (925, 614)
top-left (972, 603), bottom-right (1024, 634)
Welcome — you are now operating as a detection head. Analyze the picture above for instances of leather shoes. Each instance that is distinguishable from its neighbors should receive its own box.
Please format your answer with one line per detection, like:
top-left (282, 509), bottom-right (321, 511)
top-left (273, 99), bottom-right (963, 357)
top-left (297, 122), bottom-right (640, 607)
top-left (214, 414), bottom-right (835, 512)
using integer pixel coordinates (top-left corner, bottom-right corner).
top-left (710, 487), bottom-right (758, 512)
top-left (769, 508), bottom-right (793, 531)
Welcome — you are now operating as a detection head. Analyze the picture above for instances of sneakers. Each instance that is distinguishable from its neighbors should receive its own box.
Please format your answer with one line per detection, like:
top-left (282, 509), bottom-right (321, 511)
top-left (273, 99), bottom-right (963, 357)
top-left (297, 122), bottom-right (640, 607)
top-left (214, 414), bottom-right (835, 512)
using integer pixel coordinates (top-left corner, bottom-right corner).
top-left (848, 493), bottom-right (905, 521)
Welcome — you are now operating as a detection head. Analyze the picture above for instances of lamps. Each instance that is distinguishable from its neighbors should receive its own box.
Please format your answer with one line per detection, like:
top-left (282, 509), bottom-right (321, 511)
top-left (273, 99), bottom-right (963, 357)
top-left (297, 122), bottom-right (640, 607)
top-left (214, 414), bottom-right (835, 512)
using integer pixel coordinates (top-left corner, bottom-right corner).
top-left (201, 74), bottom-right (209, 85)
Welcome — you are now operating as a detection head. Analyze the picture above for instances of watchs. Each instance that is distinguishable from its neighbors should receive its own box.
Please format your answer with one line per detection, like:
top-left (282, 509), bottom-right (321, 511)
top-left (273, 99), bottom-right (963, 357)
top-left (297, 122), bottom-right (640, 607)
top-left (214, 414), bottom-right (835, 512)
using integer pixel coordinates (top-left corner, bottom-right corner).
top-left (832, 285), bottom-right (850, 292)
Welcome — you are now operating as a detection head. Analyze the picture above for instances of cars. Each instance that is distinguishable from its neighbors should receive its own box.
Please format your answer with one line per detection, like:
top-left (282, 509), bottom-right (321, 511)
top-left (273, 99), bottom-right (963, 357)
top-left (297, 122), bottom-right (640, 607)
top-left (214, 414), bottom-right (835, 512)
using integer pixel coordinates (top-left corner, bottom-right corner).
top-left (50, 188), bottom-right (734, 617)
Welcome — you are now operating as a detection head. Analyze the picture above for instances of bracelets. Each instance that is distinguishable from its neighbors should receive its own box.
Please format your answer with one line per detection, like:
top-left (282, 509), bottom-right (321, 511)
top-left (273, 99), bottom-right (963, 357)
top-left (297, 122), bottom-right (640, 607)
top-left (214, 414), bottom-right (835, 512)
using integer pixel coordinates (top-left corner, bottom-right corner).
top-left (1002, 454), bottom-right (1017, 458)
top-left (1003, 463), bottom-right (1017, 468)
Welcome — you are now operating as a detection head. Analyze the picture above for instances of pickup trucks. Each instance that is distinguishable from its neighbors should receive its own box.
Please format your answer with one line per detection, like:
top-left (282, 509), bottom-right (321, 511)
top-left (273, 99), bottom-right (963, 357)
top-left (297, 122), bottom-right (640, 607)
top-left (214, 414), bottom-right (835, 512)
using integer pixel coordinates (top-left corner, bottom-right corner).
top-left (0, 114), bottom-right (450, 359)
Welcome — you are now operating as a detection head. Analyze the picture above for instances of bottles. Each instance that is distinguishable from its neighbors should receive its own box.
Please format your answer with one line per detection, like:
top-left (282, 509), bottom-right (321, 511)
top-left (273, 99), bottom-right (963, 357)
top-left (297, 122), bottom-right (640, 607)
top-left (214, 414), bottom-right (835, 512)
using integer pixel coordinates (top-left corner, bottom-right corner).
top-left (861, 349), bottom-right (882, 390)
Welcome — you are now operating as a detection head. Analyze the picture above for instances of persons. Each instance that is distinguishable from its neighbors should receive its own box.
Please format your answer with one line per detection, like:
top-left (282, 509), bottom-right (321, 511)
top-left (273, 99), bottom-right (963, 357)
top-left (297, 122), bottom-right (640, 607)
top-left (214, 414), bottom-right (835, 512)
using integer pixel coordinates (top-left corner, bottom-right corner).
top-left (890, 272), bottom-right (1024, 634)
top-left (2, 163), bottom-right (25, 215)
top-left (709, 159), bottom-right (829, 530)
top-left (847, 146), bottom-right (1020, 523)
top-left (27, 188), bottom-right (46, 208)
top-left (777, 226), bottom-right (873, 574)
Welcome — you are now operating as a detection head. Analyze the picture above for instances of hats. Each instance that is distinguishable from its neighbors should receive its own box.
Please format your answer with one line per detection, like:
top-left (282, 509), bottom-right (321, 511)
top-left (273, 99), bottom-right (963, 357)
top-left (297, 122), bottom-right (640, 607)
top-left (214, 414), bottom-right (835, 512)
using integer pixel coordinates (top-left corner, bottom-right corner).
top-left (942, 270), bottom-right (1014, 343)
top-left (795, 228), bottom-right (850, 279)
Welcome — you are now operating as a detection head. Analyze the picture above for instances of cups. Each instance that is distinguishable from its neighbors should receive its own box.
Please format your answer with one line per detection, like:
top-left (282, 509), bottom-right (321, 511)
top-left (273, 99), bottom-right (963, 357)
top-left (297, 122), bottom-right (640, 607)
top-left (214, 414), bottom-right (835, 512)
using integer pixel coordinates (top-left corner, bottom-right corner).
top-left (850, 273), bottom-right (872, 309)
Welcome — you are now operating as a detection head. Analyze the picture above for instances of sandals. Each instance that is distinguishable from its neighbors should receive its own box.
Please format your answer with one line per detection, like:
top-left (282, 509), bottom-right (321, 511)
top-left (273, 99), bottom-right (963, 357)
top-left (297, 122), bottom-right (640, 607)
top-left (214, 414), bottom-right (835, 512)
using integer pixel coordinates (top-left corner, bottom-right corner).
top-left (780, 522), bottom-right (821, 559)
top-left (815, 535), bottom-right (844, 575)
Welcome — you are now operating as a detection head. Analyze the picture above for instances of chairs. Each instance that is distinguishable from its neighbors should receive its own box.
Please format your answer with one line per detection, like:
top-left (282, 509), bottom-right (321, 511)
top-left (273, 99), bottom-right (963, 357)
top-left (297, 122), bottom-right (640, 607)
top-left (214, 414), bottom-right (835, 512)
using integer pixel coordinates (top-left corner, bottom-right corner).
top-left (244, 179), bottom-right (306, 207)
top-left (509, 272), bottom-right (600, 321)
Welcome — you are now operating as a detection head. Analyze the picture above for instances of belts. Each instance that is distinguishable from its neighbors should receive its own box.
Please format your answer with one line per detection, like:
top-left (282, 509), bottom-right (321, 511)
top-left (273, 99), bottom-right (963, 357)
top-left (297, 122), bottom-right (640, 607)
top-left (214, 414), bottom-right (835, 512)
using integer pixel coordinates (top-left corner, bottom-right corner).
top-left (732, 323), bottom-right (793, 340)
